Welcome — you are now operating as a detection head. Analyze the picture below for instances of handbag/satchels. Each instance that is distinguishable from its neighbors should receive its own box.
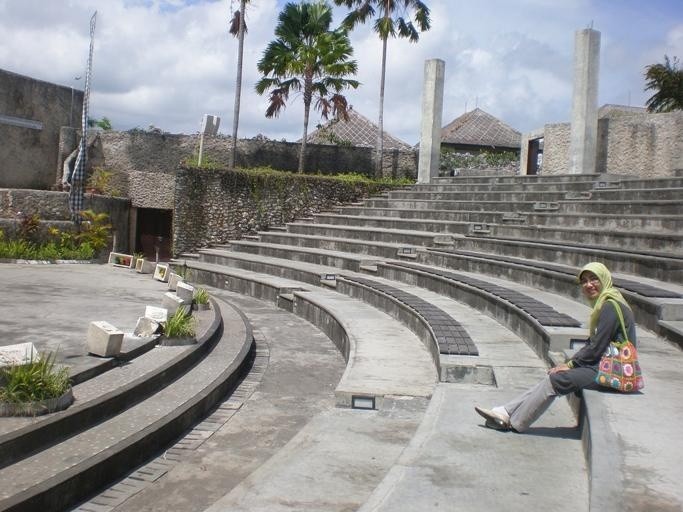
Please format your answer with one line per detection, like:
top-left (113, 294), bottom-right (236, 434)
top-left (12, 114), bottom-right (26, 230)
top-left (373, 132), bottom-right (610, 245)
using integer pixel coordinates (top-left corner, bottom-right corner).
top-left (592, 297), bottom-right (646, 394)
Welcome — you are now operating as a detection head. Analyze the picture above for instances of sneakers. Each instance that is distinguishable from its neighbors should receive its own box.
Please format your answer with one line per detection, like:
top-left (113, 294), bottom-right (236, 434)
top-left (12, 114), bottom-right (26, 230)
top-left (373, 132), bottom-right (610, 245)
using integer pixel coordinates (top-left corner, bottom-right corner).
top-left (474, 404), bottom-right (515, 430)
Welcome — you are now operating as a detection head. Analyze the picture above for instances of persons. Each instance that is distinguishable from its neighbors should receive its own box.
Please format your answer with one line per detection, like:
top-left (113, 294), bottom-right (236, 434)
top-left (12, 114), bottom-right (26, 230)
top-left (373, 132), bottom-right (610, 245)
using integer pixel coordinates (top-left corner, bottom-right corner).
top-left (478, 261), bottom-right (637, 435)
